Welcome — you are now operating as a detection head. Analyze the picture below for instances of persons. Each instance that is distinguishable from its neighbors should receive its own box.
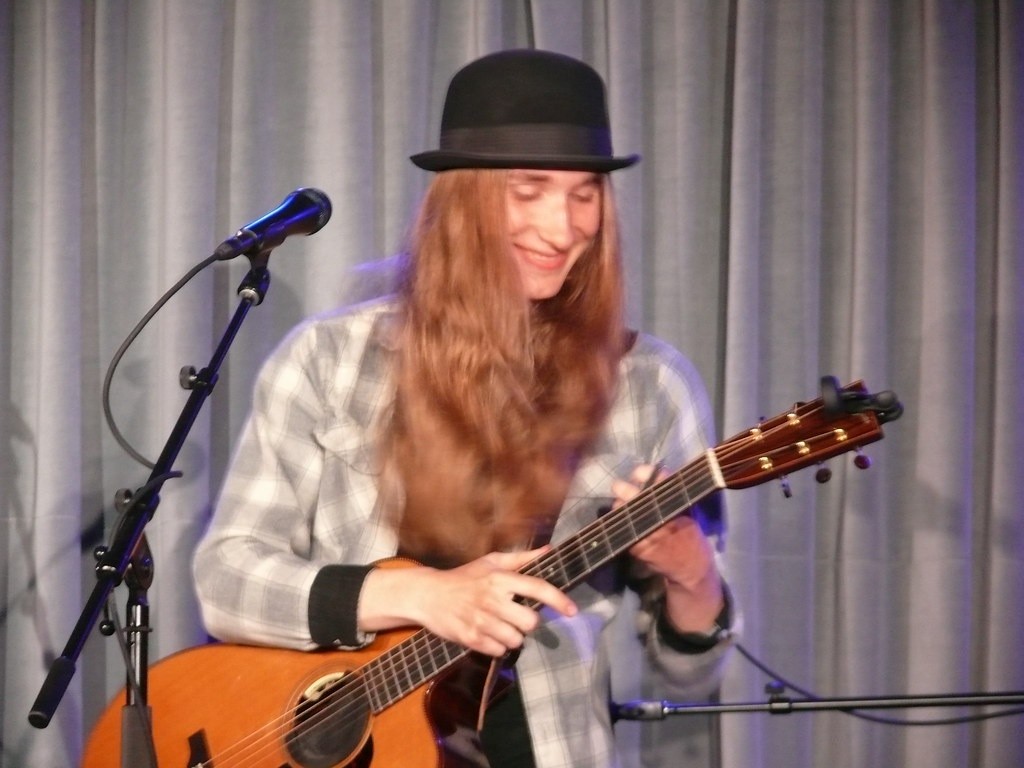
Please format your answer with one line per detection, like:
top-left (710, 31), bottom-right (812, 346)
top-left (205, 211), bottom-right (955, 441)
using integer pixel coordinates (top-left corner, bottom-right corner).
top-left (192, 50), bottom-right (744, 768)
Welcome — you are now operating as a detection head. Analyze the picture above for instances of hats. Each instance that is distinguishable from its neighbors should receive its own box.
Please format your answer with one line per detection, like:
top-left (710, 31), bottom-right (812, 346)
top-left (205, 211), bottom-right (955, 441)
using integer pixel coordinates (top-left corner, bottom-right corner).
top-left (410, 49), bottom-right (640, 174)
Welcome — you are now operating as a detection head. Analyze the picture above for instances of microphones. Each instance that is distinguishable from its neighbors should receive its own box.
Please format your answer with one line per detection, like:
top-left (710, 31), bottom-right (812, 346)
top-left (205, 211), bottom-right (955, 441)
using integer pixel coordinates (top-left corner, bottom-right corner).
top-left (216, 187), bottom-right (333, 260)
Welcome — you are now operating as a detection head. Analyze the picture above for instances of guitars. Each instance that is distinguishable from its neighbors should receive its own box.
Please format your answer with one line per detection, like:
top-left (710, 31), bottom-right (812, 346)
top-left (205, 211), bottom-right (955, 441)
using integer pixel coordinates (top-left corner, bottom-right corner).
top-left (84, 372), bottom-right (905, 768)
